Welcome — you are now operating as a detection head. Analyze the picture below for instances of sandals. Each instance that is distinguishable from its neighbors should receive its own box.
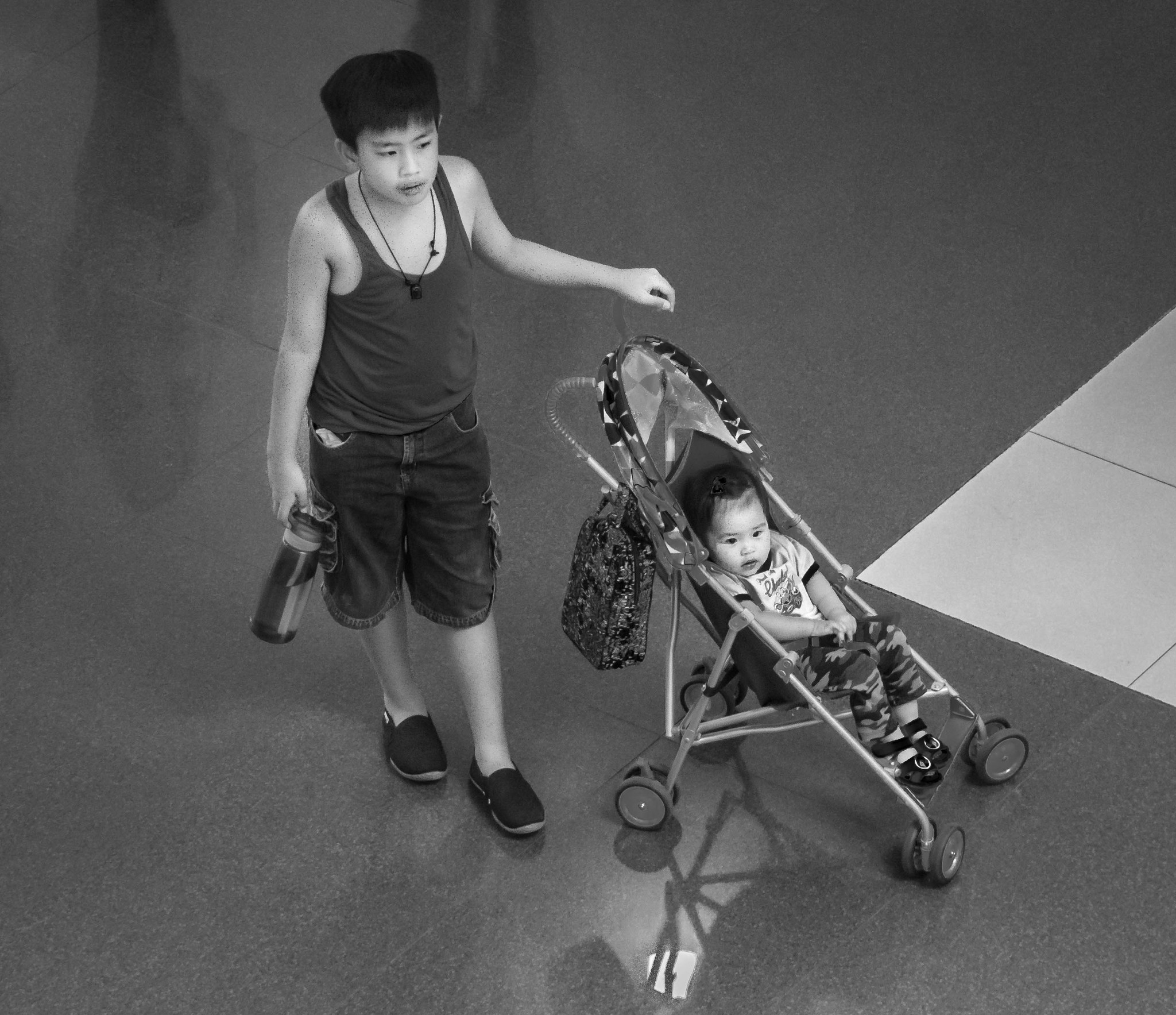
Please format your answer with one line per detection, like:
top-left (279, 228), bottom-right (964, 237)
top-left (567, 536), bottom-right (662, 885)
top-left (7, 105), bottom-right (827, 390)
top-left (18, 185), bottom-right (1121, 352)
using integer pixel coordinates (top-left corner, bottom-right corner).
top-left (870, 737), bottom-right (944, 788)
top-left (900, 717), bottom-right (952, 768)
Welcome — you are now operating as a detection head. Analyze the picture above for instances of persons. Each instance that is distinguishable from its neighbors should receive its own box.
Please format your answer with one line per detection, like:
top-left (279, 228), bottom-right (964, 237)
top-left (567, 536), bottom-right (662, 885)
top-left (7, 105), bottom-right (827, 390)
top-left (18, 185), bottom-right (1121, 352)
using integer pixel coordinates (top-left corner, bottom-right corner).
top-left (679, 460), bottom-right (953, 787)
top-left (265, 48), bottom-right (676, 836)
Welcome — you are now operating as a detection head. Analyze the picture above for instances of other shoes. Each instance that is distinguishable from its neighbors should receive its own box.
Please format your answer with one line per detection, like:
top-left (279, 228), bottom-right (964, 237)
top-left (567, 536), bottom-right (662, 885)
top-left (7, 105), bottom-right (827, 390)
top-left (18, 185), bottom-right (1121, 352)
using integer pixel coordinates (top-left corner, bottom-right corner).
top-left (383, 708), bottom-right (447, 781)
top-left (468, 757), bottom-right (546, 834)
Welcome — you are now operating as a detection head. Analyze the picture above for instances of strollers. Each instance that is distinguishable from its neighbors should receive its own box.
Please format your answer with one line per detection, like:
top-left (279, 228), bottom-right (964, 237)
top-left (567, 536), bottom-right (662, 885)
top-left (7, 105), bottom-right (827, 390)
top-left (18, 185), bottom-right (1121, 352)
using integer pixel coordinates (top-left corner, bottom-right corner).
top-left (544, 333), bottom-right (1030, 887)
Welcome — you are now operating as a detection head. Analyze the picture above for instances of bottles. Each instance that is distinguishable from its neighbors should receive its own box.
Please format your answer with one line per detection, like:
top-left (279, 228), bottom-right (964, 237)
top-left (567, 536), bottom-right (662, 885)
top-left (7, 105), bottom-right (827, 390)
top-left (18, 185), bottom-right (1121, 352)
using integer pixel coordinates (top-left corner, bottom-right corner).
top-left (247, 506), bottom-right (326, 645)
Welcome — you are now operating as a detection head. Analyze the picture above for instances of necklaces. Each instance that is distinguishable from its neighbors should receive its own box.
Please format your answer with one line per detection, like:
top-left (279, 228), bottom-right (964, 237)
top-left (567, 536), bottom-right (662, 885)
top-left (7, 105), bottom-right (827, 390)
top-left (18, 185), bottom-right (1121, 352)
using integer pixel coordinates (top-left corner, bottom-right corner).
top-left (355, 169), bottom-right (439, 300)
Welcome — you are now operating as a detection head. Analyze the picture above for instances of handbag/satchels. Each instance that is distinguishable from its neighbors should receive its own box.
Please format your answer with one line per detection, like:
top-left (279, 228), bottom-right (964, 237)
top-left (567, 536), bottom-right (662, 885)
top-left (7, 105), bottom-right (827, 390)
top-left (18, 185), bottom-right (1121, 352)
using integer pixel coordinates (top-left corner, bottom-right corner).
top-left (560, 482), bottom-right (652, 669)
